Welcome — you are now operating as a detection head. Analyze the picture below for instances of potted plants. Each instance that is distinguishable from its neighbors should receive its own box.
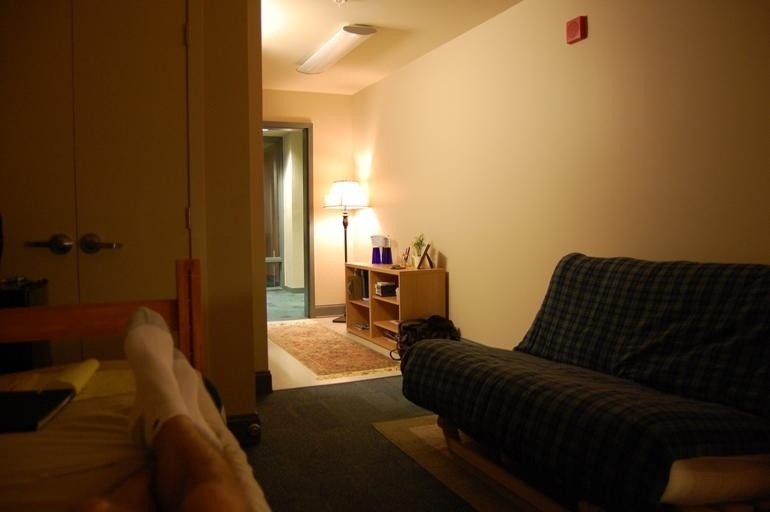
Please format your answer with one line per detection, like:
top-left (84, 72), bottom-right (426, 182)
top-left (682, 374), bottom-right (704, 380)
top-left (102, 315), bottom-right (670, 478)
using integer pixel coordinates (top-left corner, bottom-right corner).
top-left (414, 232), bottom-right (424, 269)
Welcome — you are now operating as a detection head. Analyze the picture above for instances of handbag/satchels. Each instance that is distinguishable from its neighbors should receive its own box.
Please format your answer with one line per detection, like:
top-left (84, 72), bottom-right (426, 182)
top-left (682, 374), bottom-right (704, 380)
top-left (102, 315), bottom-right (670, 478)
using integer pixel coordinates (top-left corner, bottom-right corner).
top-left (398, 315), bottom-right (459, 361)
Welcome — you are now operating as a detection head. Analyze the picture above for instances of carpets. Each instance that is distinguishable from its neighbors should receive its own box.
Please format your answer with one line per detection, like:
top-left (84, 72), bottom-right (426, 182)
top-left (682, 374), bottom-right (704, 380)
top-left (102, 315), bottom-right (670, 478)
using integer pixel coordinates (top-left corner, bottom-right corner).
top-left (269, 316), bottom-right (400, 391)
top-left (372, 410), bottom-right (584, 512)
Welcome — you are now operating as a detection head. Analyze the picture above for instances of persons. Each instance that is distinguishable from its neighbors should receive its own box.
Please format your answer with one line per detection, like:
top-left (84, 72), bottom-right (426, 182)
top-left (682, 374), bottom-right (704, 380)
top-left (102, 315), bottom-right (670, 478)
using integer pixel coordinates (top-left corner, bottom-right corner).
top-left (84, 307), bottom-right (254, 512)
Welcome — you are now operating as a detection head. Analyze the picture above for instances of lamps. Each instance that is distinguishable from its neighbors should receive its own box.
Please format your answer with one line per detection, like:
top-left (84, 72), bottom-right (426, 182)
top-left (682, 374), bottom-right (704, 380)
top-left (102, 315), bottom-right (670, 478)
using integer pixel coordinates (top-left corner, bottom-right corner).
top-left (296, 26), bottom-right (378, 74)
top-left (322, 179), bottom-right (372, 323)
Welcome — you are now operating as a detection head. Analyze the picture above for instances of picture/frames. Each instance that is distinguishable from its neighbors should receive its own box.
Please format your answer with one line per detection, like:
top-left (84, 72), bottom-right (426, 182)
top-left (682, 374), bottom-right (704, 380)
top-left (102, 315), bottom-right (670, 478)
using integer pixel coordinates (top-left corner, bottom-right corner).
top-left (416, 244), bottom-right (433, 270)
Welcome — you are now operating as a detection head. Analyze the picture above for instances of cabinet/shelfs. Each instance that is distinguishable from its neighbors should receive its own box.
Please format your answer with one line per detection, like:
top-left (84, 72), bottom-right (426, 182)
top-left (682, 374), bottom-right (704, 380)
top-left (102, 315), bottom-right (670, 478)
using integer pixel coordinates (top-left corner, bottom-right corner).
top-left (345, 263), bottom-right (446, 355)
top-left (0, 0), bottom-right (257, 415)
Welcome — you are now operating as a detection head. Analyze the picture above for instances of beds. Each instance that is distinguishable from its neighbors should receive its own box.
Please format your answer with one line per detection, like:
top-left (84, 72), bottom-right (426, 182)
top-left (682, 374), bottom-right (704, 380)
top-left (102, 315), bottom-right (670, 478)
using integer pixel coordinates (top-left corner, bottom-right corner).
top-left (0, 258), bottom-right (273, 512)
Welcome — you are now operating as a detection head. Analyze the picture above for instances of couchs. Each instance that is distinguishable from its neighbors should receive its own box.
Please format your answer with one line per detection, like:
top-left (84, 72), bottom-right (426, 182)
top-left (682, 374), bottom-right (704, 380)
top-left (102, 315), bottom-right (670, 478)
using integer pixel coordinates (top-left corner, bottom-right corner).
top-left (402, 253), bottom-right (770, 512)
